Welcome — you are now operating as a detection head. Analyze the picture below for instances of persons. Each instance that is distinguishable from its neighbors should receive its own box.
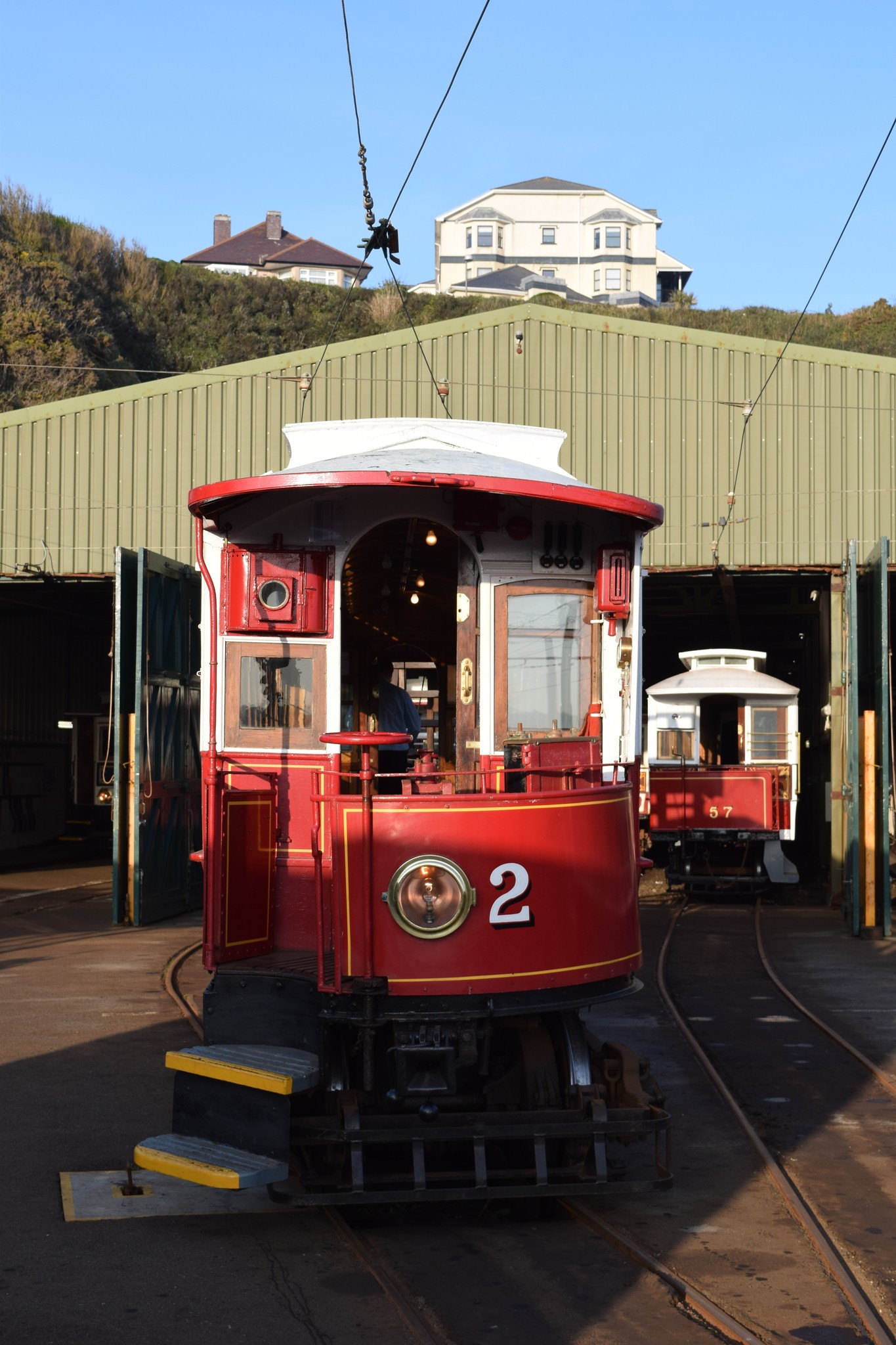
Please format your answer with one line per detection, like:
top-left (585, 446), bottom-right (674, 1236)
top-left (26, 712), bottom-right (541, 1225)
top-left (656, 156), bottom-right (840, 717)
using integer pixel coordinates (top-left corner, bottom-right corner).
top-left (342, 660), bottom-right (421, 794)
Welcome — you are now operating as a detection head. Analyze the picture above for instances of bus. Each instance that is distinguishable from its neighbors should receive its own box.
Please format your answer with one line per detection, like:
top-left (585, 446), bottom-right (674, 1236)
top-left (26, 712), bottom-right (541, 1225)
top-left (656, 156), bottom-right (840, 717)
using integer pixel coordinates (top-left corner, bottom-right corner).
top-left (644, 646), bottom-right (804, 886)
top-left (128, 410), bottom-right (674, 1218)
